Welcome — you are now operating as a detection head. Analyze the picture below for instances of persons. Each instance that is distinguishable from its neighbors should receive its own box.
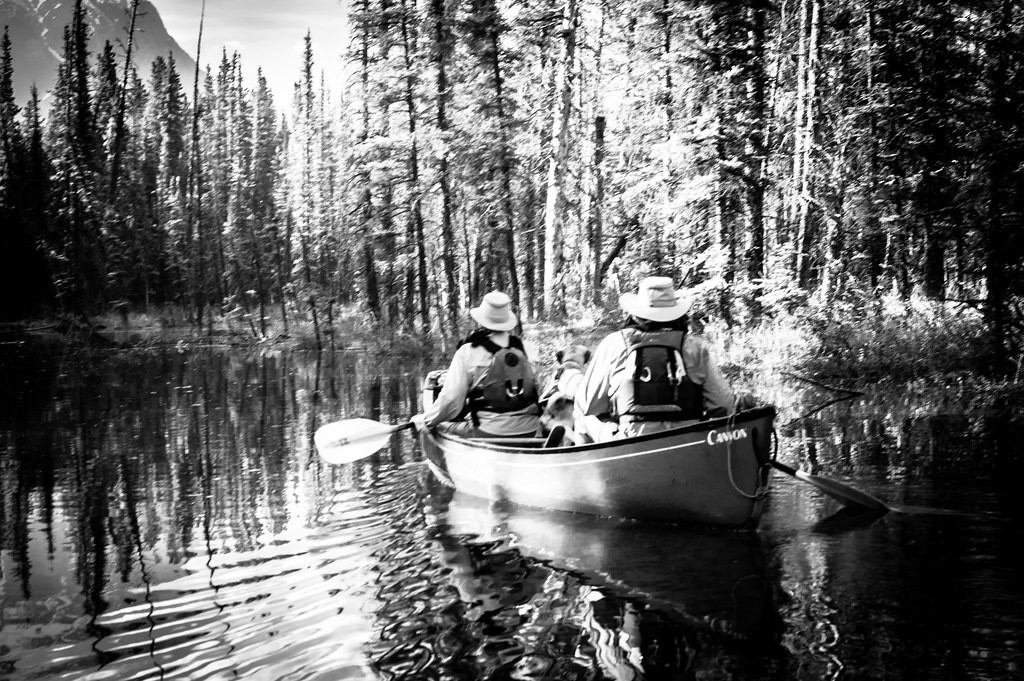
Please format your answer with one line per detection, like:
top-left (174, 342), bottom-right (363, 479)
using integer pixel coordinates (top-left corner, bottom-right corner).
top-left (573, 276), bottom-right (734, 445)
top-left (407, 292), bottom-right (540, 437)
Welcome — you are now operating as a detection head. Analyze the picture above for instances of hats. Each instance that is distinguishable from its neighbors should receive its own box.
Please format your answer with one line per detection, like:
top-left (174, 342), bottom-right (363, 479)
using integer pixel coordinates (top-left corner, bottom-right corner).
top-left (618, 276), bottom-right (693, 322)
top-left (469, 291), bottom-right (517, 331)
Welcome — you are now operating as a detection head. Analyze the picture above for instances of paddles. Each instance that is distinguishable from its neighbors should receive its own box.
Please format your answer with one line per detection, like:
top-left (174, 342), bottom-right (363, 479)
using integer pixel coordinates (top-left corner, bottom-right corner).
top-left (770, 458), bottom-right (893, 512)
top-left (314, 418), bottom-right (415, 468)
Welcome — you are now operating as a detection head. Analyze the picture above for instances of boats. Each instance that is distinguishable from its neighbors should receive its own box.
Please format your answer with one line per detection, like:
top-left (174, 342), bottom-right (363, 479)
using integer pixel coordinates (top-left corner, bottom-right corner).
top-left (447, 487), bottom-right (780, 649)
top-left (421, 368), bottom-right (777, 537)
